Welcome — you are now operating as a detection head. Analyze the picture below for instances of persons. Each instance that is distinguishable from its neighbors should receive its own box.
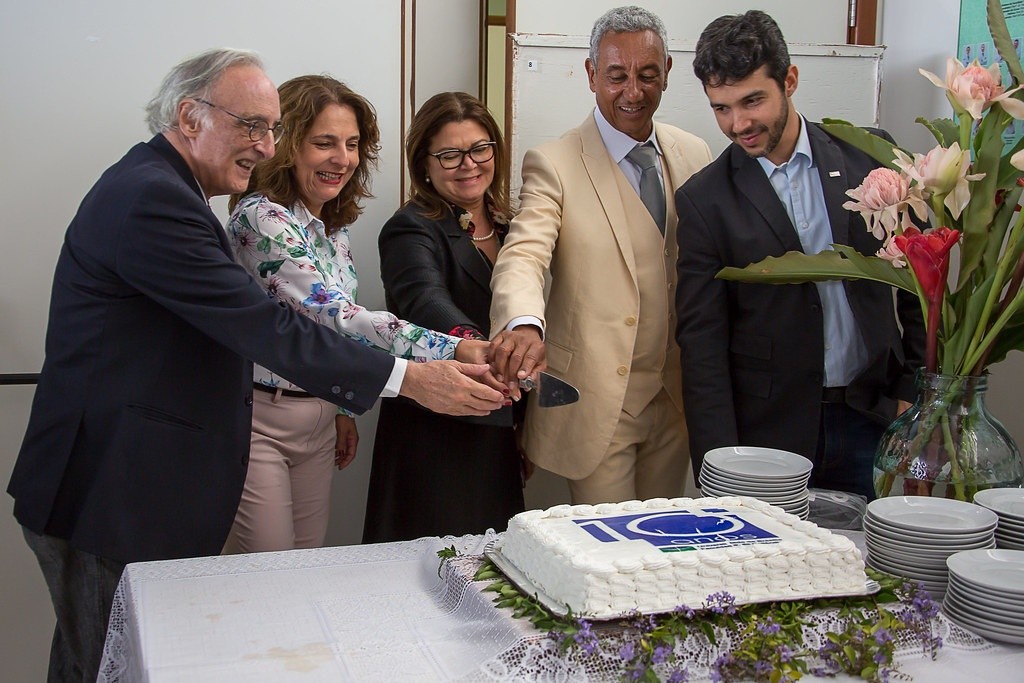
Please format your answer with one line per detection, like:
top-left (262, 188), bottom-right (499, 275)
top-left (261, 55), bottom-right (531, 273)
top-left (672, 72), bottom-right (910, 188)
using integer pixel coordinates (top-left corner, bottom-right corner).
top-left (5, 45), bottom-right (515, 683)
top-left (486, 6), bottom-right (720, 511)
top-left (670, 8), bottom-right (930, 514)
top-left (220, 72), bottom-right (513, 553)
top-left (362, 89), bottom-right (527, 544)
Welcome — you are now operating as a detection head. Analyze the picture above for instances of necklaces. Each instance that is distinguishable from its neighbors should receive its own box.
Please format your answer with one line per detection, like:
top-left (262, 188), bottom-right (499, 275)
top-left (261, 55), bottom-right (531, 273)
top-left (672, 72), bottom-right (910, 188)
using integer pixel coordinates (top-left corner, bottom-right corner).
top-left (474, 228), bottom-right (495, 241)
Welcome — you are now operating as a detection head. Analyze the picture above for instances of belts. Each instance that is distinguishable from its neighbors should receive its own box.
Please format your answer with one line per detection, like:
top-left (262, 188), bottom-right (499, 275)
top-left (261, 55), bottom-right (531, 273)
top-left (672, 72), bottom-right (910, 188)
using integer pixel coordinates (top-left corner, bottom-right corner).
top-left (254, 382), bottom-right (316, 399)
top-left (820, 385), bottom-right (849, 404)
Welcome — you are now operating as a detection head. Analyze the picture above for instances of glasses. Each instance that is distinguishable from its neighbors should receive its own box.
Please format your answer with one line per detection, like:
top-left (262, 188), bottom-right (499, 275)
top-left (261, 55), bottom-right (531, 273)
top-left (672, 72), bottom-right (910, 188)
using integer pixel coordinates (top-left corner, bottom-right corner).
top-left (426, 137), bottom-right (498, 170)
top-left (193, 95), bottom-right (285, 145)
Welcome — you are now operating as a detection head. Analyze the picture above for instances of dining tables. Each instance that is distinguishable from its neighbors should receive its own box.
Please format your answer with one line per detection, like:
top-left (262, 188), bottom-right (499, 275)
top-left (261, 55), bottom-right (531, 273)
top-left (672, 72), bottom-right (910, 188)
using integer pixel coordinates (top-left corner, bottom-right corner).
top-left (93, 529), bottom-right (1023, 681)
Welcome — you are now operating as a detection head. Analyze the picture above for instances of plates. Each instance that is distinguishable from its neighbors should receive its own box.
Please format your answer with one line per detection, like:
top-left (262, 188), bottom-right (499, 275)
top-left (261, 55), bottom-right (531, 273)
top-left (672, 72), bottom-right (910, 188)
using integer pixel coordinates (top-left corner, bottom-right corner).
top-left (861, 496), bottom-right (999, 601)
top-left (696, 445), bottom-right (815, 523)
top-left (940, 548), bottom-right (1024, 647)
top-left (972, 487), bottom-right (1024, 550)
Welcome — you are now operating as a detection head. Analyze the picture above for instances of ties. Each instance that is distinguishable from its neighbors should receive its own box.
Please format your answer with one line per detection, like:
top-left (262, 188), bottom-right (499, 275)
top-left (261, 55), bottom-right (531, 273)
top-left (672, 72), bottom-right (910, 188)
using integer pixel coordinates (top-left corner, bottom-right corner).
top-left (632, 145), bottom-right (666, 232)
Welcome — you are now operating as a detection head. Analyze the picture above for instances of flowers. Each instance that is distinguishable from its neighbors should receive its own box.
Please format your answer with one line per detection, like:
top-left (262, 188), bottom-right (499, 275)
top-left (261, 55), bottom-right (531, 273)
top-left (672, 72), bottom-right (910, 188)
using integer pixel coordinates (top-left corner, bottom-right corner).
top-left (714, 0), bottom-right (1023, 502)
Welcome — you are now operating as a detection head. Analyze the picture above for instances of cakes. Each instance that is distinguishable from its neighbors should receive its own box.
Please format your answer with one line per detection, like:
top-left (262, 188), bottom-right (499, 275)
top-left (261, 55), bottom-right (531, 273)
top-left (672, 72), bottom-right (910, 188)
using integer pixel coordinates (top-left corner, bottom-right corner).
top-left (499, 495), bottom-right (866, 618)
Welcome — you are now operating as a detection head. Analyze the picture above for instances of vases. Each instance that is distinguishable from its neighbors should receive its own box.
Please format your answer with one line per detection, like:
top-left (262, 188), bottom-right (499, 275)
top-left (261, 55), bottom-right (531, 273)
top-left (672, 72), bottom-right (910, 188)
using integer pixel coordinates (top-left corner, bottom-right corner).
top-left (871, 370), bottom-right (1022, 503)
